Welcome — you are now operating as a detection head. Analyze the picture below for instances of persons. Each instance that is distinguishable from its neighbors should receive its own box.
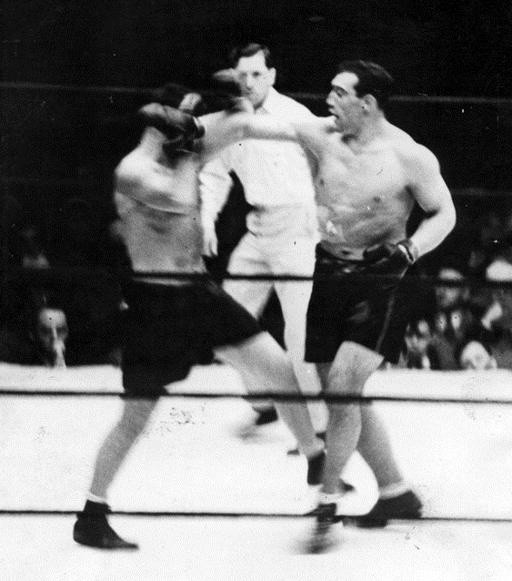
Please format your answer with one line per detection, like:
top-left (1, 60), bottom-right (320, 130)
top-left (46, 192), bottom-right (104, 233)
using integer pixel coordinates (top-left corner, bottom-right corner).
top-left (200, 42), bottom-right (330, 455)
top-left (198, 57), bottom-right (457, 554)
top-left (31, 295), bottom-right (69, 367)
top-left (75, 85), bottom-right (353, 550)
top-left (384, 251), bottom-right (511, 370)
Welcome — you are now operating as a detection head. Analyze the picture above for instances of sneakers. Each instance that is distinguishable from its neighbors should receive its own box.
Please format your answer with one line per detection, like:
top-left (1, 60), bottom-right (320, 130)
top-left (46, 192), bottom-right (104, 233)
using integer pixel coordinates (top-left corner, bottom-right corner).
top-left (303, 448), bottom-right (424, 555)
top-left (72, 499), bottom-right (139, 551)
top-left (242, 404), bottom-right (280, 438)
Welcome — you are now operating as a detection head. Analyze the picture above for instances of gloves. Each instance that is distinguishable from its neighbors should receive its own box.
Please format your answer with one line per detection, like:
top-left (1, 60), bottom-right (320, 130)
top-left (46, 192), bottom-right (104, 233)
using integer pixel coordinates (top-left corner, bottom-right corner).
top-left (136, 92), bottom-right (206, 171)
top-left (362, 241), bottom-right (418, 293)
top-left (212, 69), bottom-right (244, 114)
